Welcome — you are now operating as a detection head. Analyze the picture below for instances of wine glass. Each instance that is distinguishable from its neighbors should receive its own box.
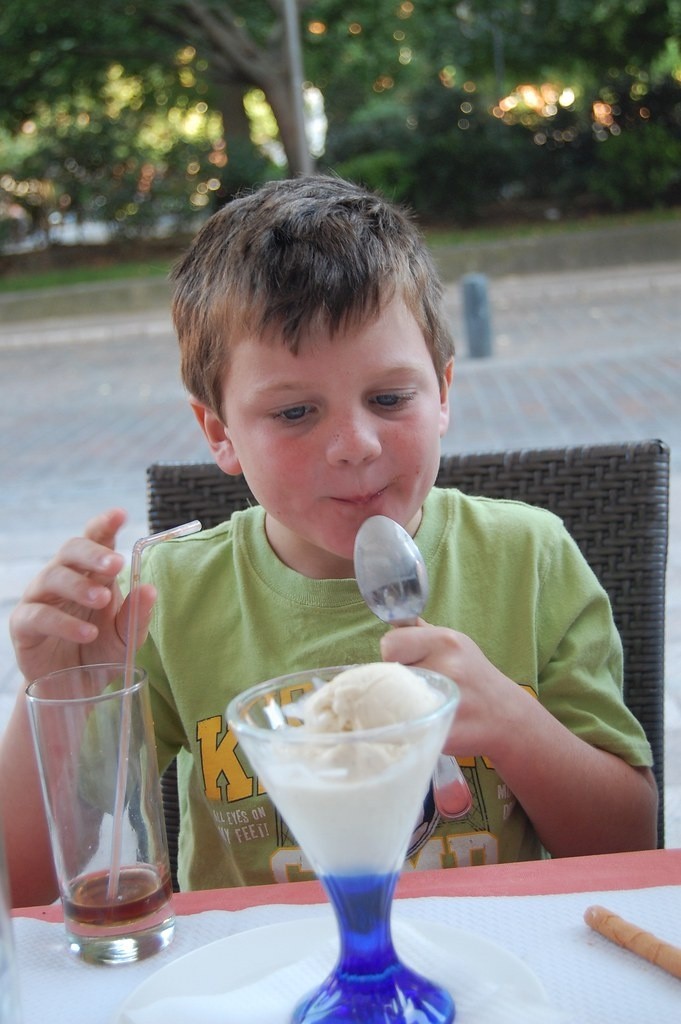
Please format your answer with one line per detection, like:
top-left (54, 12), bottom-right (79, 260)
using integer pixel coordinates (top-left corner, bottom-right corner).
top-left (223, 662), bottom-right (459, 1024)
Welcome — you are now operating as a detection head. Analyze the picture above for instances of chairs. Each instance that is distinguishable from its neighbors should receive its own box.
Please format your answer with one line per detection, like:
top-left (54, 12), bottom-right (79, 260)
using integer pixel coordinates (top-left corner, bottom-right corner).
top-left (128, 436), bottom-right (669, 894)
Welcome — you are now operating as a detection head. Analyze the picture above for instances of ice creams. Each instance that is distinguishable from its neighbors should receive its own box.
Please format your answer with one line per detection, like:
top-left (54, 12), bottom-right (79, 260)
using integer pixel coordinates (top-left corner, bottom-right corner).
top-left (256, 660), bottom-right (447, 878)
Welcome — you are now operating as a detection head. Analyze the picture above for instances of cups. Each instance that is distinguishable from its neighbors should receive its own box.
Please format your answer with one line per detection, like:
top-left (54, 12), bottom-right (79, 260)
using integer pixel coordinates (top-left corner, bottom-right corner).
top-left (24, 665), bottom-right (176, 968)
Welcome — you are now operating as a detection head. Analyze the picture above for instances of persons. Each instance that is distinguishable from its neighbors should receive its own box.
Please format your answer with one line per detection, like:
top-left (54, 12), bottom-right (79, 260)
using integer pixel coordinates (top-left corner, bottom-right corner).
top-left (0, 174), bottom-right (661, 908)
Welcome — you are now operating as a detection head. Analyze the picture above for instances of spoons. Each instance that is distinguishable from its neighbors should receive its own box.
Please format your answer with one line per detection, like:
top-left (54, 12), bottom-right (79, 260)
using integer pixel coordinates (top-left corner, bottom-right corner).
top-left (352, 514), bottom-right (472, 819)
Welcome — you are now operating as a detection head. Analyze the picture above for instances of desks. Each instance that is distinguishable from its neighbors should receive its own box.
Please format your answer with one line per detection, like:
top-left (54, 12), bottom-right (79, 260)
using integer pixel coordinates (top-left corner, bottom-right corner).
top-left (0, 851), bottom-right (681, 1024)
